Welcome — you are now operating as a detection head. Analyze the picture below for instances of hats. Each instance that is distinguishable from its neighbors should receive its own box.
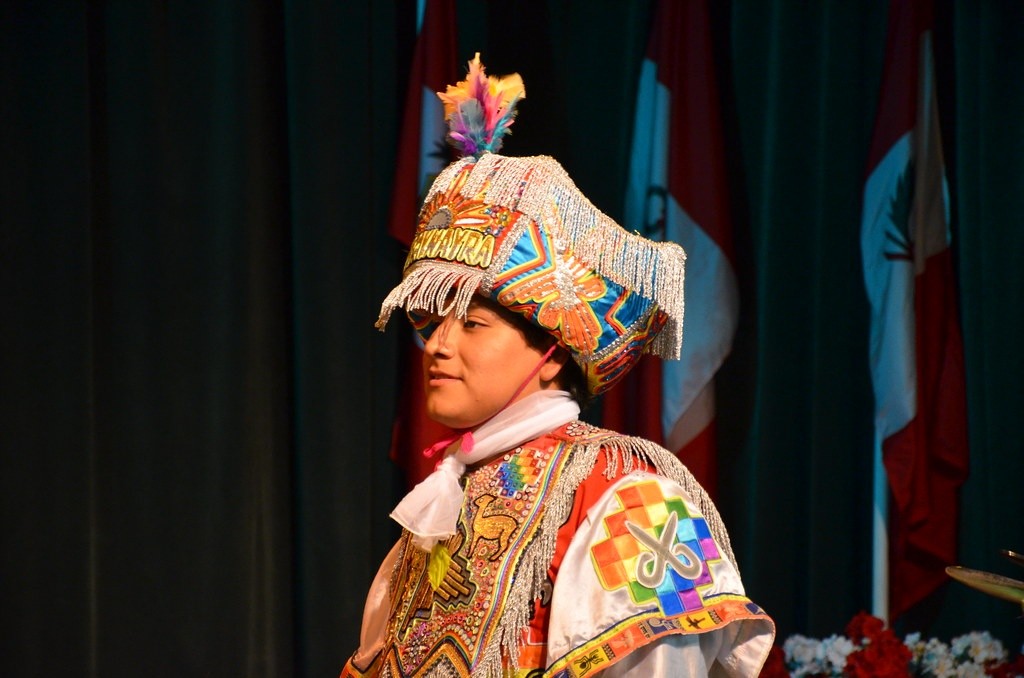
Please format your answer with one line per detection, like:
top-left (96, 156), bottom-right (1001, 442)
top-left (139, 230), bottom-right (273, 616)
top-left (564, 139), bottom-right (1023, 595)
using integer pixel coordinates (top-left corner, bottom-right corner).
top-left (376, 52), bottom-right (686, 399)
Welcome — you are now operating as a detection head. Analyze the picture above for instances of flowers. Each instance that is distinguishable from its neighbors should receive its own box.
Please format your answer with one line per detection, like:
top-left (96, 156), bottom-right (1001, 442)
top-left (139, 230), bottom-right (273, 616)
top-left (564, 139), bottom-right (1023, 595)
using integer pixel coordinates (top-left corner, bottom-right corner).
top-left (759, 612), bottom-right (1024, 678)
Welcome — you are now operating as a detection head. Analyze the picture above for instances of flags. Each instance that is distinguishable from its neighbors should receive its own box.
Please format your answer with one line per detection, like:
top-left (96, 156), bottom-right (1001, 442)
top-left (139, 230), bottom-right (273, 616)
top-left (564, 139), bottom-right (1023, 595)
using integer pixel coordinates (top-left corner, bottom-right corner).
top-left (860, 0), bottom-right (971, 625)
top-left (600, 1), bottom-right (742, 503)
top-left (389, 0), bottom-right (461, 249)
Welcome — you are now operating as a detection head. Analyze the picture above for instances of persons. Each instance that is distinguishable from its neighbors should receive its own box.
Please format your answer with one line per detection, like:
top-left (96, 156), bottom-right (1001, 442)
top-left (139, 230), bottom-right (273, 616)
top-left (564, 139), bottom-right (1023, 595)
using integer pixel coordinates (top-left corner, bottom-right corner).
top-left (340, 142), bottom-right (776, 677)
